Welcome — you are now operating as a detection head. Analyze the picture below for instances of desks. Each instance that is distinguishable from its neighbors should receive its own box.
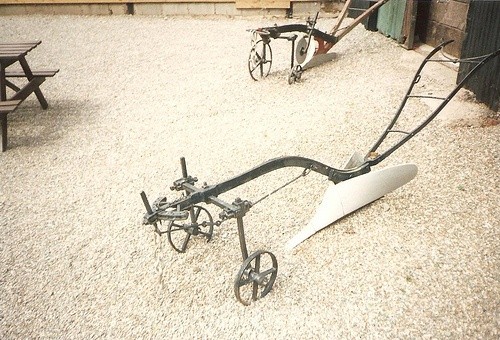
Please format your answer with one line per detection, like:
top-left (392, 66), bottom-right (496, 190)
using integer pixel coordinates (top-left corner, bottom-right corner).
top-left (0, 39), bottom-right (50, 153)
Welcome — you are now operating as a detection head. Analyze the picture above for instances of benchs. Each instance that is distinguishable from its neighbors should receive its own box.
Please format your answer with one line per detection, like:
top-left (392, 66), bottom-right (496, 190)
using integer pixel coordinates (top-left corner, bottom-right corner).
top-left (0, 99), bottom-right (23, 119)
top-left (5, 68), bottom-right (60, 86)
